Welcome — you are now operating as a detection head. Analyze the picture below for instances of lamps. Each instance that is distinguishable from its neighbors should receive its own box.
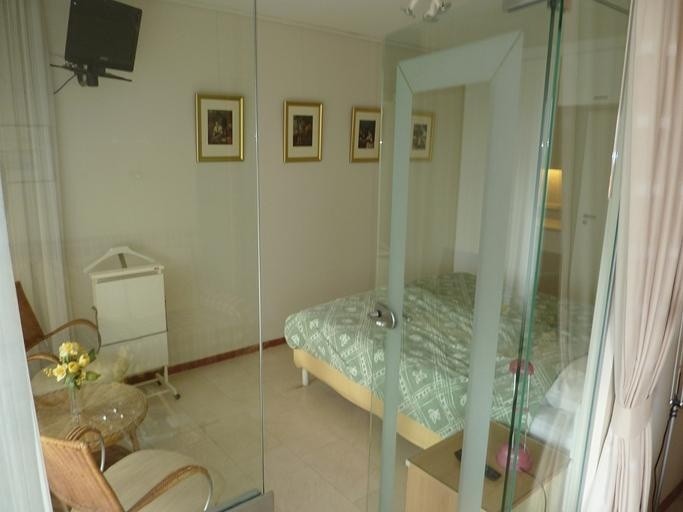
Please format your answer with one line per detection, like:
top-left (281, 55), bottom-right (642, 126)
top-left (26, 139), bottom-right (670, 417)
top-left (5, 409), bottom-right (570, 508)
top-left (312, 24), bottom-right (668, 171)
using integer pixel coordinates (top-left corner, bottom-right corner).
top-left (496, 359), bottom-right (534, 474)
top-left (399, 0), bottom-right (452, 22)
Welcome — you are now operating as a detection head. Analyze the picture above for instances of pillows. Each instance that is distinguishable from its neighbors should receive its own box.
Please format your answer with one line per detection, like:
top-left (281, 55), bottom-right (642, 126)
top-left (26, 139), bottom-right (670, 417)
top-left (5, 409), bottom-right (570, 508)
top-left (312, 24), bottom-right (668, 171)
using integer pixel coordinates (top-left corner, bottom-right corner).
top-left (529, 408), bottom-right (577, 450)
top-left (545, 355), bottom-right (588, 413)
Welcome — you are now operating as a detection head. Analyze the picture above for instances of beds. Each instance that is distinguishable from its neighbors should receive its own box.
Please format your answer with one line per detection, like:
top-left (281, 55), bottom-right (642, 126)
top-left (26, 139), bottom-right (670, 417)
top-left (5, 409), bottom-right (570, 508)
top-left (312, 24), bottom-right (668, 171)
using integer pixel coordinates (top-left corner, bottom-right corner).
top-left (283, 271), bottom-right (595, 450)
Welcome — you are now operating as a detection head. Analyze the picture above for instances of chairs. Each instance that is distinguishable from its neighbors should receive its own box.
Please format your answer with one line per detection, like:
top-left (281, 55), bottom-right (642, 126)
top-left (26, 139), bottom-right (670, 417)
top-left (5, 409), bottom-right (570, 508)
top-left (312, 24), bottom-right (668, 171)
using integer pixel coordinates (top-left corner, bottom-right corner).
top-left (38, 424), bottom-right (216, 512)
top-left (14, 280), bottom-right (130, 398)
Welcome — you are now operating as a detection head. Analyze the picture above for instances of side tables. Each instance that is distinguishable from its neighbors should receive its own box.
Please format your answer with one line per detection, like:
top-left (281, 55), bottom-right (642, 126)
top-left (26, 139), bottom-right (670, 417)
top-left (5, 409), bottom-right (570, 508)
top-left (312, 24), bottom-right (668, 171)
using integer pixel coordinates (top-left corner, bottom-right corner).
top-left (35, 382), bottom-right (149, 469)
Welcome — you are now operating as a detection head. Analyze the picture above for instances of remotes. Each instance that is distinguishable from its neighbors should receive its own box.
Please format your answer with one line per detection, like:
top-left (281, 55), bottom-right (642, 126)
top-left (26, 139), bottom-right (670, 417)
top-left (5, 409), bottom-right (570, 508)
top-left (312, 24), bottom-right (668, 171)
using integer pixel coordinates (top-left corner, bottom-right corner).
top-left (455, 449), bottom-right (501, 480)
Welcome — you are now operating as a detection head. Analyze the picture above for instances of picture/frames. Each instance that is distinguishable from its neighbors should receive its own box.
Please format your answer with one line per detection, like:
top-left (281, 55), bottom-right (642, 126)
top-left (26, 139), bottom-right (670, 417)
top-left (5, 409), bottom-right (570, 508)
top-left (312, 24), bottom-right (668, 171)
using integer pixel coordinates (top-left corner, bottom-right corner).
top-left (194, 90), bottom-right (245, 164)
top-left (408, 108), bottom-right (435, 161)
top-left (281, 98), bottom-right (324, 164)
top-left (348, 104), bottom-right (384, 164)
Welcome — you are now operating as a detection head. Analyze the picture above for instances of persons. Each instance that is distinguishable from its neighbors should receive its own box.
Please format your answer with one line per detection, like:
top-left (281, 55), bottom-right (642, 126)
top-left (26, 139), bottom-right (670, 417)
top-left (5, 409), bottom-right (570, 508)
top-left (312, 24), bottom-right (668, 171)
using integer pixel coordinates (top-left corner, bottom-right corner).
top-left (210, 119), bottom-right (224, 144)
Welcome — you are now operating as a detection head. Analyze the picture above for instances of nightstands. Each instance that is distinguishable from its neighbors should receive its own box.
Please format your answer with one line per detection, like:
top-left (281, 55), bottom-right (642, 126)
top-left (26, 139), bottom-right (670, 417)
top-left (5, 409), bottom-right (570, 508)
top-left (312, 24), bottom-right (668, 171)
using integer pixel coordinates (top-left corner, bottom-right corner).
top-left (404, 420), bottom-right (573, 512)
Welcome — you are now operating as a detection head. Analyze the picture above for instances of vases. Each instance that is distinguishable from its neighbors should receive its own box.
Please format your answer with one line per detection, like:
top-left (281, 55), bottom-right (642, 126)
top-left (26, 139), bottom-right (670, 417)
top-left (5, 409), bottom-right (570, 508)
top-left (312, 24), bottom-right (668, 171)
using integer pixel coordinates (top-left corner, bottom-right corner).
top-left (68, 384), bottom-right (82, 426)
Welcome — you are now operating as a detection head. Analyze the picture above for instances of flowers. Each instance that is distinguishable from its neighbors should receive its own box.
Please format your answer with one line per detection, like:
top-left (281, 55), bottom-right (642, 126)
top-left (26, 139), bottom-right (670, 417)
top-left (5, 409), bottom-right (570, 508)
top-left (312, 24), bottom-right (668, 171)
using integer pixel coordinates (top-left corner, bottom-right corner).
top-left (40, 341), bottom-right (102, 391)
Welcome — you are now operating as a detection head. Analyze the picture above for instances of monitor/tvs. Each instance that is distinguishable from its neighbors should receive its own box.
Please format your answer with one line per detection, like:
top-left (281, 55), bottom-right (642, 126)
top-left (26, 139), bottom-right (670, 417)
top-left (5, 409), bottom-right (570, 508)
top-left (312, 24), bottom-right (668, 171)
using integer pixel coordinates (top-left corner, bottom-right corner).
top-left (65, 1), bottom-right (142, 75)
